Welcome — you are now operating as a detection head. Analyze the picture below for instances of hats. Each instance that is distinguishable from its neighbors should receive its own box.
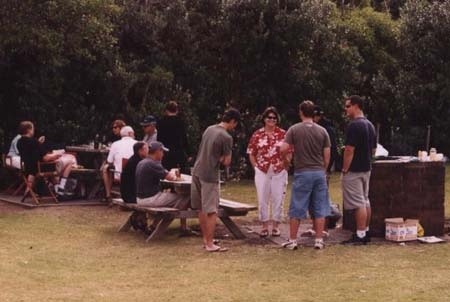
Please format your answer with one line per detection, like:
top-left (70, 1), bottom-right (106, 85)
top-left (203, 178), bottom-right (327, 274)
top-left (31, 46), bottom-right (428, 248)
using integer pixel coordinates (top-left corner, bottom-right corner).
top-left (141, 115), bottom-right (157, 125)
top-left (148, 142), bottom-right (169, 153)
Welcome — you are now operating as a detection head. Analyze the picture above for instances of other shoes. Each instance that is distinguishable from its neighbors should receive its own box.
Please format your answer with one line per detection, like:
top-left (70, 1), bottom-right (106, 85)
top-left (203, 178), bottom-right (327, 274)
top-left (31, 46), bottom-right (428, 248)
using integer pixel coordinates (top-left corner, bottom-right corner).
top-left (54, 184), bottom-right (64, 202)
top-left (101, 197), bottom-right (108, 202)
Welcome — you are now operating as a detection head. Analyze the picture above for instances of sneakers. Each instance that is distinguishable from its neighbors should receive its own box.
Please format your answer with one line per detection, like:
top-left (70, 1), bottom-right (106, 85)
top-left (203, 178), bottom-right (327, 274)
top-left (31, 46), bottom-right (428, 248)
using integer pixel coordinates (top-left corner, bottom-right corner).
top-left (282, 240), bottom-right (298, 249)
top-left (315, 240), bottom-right (325, 249)
top-left (340, 231), bottom-right (371, 247)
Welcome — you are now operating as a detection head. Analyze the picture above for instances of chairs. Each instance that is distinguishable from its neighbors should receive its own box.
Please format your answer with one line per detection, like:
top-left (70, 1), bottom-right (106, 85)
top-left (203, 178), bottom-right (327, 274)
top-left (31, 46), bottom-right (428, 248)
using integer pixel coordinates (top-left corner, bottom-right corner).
top-left (2, 154), bottom-right (60, 204)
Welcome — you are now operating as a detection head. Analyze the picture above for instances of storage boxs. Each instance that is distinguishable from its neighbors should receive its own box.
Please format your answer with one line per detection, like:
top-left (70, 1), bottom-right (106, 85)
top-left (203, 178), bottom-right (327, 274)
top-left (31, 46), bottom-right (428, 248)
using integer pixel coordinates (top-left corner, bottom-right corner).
top-left (384, 217), bottom-right (420, 242)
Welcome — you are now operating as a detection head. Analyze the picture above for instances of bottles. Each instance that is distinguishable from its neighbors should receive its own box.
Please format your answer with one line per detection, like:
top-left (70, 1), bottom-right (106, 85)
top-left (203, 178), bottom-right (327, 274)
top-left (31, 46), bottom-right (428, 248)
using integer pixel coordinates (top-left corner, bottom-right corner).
top-left (94, 133), bottom-right (99, 149)
top-left (430, 148), bottom-right (437, 159)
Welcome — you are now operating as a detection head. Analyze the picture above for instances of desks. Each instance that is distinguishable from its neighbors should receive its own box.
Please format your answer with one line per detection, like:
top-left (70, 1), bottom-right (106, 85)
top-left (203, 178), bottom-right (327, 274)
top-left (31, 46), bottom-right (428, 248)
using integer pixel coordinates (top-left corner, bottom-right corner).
top-left (117, 174), bottom-right (246, 242)
top-left (369, 156), bottom-right (446, 238)
top-left (67, 145), bottom-right (110, 200)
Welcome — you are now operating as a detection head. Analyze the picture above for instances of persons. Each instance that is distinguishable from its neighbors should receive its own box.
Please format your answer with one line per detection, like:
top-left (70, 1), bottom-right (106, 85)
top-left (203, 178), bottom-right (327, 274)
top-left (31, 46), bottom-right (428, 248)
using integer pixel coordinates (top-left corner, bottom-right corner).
top-left (156, 100), bottom-right (188, 173)
top-left (135, 141), bottom-right (183, 237)
top-left (100, 119), bottom-right (126, 202)
top-left (17, 121), bottom-right (72, 197)
top-left (107, 126), bottom-right (138, 184)
top-left (279, 100), bottom-right (331, 249)
top-left (308, 105), bottom-right (342, 229)
top-left (119, 141), bottom-right (147, 230)
top-left (246, 106), bottom-right (294, 237)
top-left (340, 95), bottom-right (377, 246)
top-left (139, 114), bottom-right (157, 146)
top-left (191, 108), bottom-right (240, 251)
top-left (6, 122), bottom-right (24, 194)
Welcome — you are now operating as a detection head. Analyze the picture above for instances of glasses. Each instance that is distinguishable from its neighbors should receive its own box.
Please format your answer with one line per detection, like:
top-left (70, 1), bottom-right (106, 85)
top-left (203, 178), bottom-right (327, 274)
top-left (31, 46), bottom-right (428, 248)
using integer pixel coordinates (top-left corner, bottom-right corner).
top-left (111, 125), bottom-right (119, 129)
top-left (345, 104), bottom-right (351, 108)
top-left (267, 116), bottom-right (278, 120)
top-left (142, 125), bottom-right (149, 128)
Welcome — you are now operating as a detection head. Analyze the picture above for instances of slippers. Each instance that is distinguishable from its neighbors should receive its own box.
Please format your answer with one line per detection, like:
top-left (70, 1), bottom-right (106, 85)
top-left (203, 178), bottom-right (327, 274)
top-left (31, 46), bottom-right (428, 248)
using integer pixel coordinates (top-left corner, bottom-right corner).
top-left (210, 247), bottom-right (229, 253)
top-left (272, 228), bottom-right (281, 236)
top-left (214, 239), bottom-right (221, 244)
top-left (259, 229), bottom-right (269, 237)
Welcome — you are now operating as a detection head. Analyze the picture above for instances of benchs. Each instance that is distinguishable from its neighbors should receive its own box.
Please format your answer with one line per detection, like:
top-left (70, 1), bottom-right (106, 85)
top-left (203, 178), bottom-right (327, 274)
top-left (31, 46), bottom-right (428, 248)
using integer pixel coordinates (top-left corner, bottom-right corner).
top-left (219, 198), bottom-right (258, 212)
top-left (112, 198), bottom-right (179, 215)
